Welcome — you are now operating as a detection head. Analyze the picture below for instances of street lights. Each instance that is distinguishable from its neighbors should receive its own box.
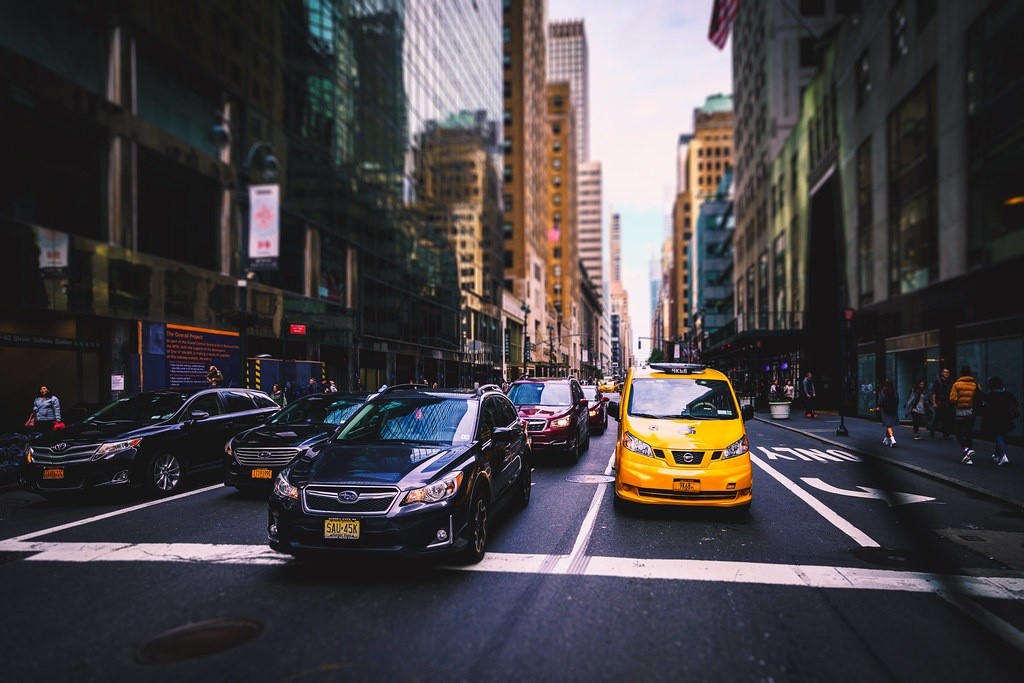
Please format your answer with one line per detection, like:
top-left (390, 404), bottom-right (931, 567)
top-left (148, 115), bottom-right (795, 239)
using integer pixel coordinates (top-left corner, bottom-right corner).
top-left (579, 343), bottom-right (586, 380)
top-left (546, 321), bottom-right (554, 377)
top-left (519, 300), bottom-right (532, 381)
top-left (206, 122), bottom-right (280, 389)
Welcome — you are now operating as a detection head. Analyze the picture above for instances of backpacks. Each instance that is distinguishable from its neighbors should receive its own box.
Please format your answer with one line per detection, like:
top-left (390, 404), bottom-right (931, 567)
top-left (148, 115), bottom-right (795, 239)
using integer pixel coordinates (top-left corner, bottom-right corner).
top-left (881, 392), bottom-right (899, 416)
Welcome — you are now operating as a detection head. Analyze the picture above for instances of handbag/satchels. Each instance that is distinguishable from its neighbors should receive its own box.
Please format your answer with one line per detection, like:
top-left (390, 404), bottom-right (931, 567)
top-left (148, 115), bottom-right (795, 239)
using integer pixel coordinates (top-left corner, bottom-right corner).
top-left (52, 421), bottom-right (66, 431)
top-left (973, 382), bottom-right (988, 417)
top-left (24, 411), bottom-right (35, 428)
top-left (1001, 388), bottom-right (1020, 420)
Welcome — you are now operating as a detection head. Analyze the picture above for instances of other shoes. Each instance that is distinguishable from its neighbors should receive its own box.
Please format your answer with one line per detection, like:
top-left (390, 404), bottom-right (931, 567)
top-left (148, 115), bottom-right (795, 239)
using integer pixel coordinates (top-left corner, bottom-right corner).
top-left (913, 433), bottom-right (923, 440)
top-left (883, 436), bottom-right (890, 446)
top-left (888, 436), bottom-right (897, 448)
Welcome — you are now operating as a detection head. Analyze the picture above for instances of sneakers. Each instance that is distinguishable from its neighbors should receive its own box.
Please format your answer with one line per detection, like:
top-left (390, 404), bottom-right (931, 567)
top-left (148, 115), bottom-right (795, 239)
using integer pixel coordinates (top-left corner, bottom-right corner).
top-left (960, 449), bottom-right (976, 465)
top-left (991, 453), bottom-right (1009, 466)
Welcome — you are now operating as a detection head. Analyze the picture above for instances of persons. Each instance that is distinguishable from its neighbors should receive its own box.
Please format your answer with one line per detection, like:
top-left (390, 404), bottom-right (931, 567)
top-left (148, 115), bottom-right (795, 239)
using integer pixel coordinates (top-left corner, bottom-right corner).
top-left (33, 385), bottom-right (61, 433)
top-left (802, 371), bottom-right (817, 418)
top-left (903, 365), bottom-right (1019, 465)
top-left (208, 381), bottom-right (222, 389)
top-left (861, 377), bottom-right (873, 412)
top-left (407, 376), bottom-right (416, 384)
top-left (306, 377), bottom-right (338, 395)
top-left (770, 378), bottom-right (780, 402)
top-left (270, 384), bottom-right (287, 407)
top-left (206, 366), bottom-right (223, 382)
top-left (783, 380), bottom-right (795, 411)
top-left (579, 376), bottom-right (598, 390)
top-left (878, 376), bottom-right (900, 448)
top-left (353, 378), bottom-right (364, 391)
top-left (422, 378), bottom-right (438, 389)
top-left (500, 378), bottom-right (513, 392)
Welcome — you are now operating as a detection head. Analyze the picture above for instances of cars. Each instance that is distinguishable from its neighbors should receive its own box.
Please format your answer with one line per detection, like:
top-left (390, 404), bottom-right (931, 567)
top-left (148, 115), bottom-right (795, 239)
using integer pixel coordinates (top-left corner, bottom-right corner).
top-left (579, 383), bottom-right (610, 436)
top-left (221, 391), bottom-right (370, 499)
top-left (593, 378), bottom-right (624, 396)
top-left (18, 387), bottom-right (282, 498)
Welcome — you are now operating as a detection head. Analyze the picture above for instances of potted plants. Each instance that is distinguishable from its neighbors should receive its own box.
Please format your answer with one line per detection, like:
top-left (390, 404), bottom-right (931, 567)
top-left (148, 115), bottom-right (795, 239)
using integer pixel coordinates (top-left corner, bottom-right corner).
top-left (768, 390), bottom-right (794, 418)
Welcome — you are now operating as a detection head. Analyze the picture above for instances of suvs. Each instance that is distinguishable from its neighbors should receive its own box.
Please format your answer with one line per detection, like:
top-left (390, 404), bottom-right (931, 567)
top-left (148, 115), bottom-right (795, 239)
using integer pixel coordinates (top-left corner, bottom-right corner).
top-left (263, 382), bottom-right (534, 567)
top-left (504, 374), bottom-right (591, 468)
top-left (604, 360), bottom-right (755, 512)
